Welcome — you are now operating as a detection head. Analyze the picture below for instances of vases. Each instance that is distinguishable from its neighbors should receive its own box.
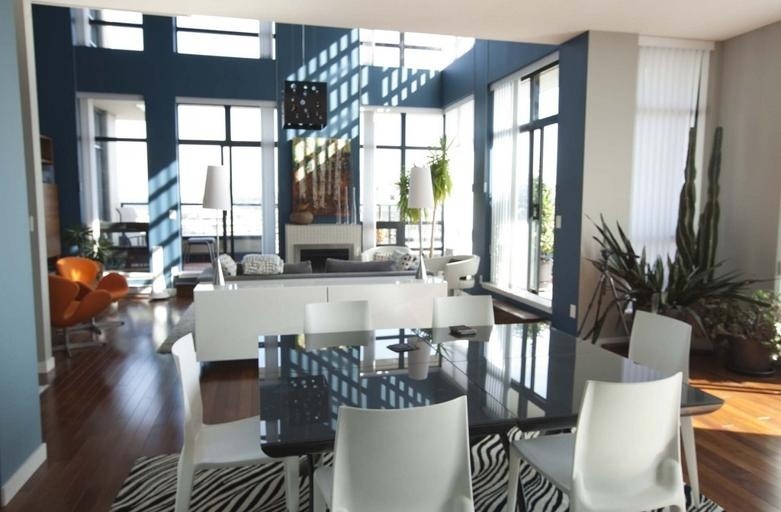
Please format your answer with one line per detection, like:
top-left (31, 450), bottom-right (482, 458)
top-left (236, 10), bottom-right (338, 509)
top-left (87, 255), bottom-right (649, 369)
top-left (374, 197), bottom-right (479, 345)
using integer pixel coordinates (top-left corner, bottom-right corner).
top-left (290, 210), bottom-right (314, 225)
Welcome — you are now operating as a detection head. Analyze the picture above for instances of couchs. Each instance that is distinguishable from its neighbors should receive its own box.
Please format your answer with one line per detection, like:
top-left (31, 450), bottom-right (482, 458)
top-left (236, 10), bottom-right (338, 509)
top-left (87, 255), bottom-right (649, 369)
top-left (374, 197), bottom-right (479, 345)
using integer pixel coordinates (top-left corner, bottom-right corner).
top-left (193, 266), bottom-right (449, 363)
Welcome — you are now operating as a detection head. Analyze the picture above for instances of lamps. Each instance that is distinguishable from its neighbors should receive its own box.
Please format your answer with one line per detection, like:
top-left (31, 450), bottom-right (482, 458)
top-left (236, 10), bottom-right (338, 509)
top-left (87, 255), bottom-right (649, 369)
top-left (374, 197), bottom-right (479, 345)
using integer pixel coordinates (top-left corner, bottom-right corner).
top-left (203, 166), bottom-right (232, 285)
top-left (408, 167), bottom-right (436, 279)
top-left (408, 327), bottom-right (432, 380)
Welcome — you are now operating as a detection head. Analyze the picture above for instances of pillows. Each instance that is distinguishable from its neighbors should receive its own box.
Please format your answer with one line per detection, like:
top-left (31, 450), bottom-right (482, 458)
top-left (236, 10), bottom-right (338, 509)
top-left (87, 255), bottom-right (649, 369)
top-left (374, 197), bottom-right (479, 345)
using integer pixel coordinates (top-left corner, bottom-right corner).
top-left (392, 249), bottom-right (405, 263)
top-left (398, 253), bottom-right (419, 270)
top-left (326, 257), bottom-right (405, 273)
top-left (237, 260), bottom-right (314, 274)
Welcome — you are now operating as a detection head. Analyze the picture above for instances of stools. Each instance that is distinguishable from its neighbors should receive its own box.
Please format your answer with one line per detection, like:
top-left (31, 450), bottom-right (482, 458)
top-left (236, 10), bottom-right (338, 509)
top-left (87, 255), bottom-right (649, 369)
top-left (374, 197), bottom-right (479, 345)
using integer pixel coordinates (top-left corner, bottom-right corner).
top-left (184, 237), bottom-right (218, 263)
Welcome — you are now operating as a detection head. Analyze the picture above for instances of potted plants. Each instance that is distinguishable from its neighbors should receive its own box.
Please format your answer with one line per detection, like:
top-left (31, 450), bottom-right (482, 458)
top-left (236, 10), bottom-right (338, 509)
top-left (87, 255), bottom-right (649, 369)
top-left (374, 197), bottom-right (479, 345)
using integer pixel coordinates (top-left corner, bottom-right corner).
top-left (715, 289), bottom-right (781, 377)
top-left (533, 176), bottom-right (554, 287)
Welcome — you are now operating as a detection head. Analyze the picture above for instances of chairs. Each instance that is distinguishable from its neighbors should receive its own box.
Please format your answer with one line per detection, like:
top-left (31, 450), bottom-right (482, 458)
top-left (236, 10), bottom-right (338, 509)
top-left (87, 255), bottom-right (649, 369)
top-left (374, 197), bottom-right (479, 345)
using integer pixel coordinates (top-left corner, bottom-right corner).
top-left (629, 310), bottom-right (700, 508)
top-left (305, 299), bottom-right (370, 333)
top-left (361, 246), bottom-right (413, 261)
top-left (48, 273), bottom-right (114, 358)
top-left (171, 333), bottom-right (300, 512)
top-left (313, 395), bottom-right (476, 512)
top-left (425, 254), bottom-right (481, 296)
top-left (56, 256), bottom-right (130, 335)
top-left (116, 206), bottom-right (147, 267)
top-left (507, 372), bottom-right (687, 511)
top-left (432, 295), bottom-right (495, 328)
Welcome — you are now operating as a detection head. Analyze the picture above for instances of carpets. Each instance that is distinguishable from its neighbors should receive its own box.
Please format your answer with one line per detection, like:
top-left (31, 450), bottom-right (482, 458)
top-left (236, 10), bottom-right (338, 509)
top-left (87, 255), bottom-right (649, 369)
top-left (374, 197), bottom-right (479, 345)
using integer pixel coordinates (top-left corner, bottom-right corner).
top-left (157, 300), bottom-right (194, 353)
top-left (109, 425), bottom-right (726, 511)
